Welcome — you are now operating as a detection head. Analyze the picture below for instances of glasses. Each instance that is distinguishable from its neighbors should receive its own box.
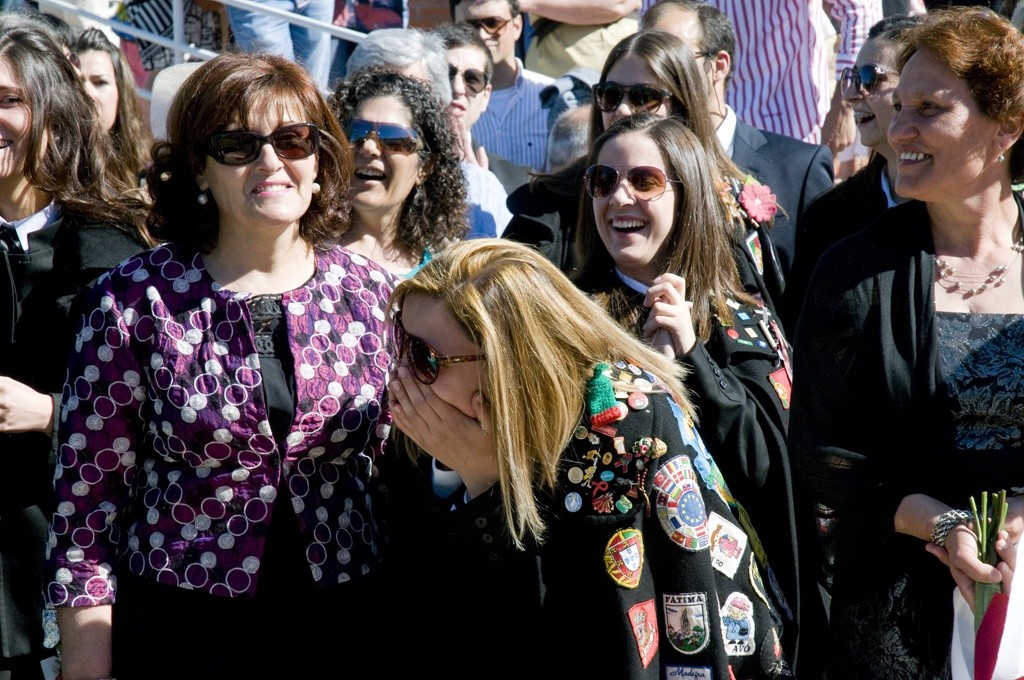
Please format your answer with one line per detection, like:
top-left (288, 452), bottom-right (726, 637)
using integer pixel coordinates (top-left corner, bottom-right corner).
top-left (196, 124), bottom-right (320, 167)
top-left (340, 117), bottom-right (426, 154)
top-left (591, 81), bottom-right (675, 117)
top-left (390, 310), bottom-right (487, 386)
top-left (840, 64), bottom-right (897, 100)
top-left (447, 62), bottom-right (488, 94)
top-left (583, 164), bottom-right (683, 202)
top-left (467, 16), bottom-right (515, 34)
top-left (66, 52), bottom-right (81, 71)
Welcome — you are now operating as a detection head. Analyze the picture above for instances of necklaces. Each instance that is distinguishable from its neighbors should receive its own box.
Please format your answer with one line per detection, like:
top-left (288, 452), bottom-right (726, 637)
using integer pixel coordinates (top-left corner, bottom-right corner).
top-left (935, 231), bottom-right (1024, 295)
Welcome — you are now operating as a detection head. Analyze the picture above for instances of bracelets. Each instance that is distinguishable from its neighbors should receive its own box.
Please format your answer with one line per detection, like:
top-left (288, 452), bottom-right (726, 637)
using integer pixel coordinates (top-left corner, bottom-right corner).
top-left (932, 509), bottom-right (976, 548)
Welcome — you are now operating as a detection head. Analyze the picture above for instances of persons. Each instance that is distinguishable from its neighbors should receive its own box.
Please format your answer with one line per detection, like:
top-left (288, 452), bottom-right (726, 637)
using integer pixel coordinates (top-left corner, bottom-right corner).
top-left (0, 0), bottom-right (1024, 680)
